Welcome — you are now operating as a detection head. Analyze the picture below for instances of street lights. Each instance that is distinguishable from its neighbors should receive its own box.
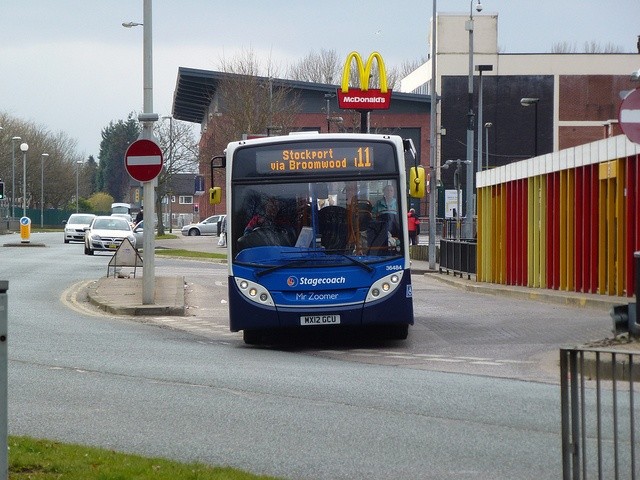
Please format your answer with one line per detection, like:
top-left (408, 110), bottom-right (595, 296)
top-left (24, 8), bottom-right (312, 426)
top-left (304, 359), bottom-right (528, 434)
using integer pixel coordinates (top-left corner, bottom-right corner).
top-left (20, 142), bottom-right (29, 217)
top-left (12, 137), bottom-right (22, 221)
top-left (76, 161), bottom-right (83, 213)
top-left (162, 115), bottom-right (172, 233)
top-left (41, 153), bottom-right (50, 228)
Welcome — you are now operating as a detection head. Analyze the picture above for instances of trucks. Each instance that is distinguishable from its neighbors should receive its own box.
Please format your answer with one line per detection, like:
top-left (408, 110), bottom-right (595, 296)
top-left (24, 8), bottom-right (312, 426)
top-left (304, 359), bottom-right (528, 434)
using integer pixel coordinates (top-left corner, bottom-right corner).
top-left (110, 202), bottom-right (131, 214)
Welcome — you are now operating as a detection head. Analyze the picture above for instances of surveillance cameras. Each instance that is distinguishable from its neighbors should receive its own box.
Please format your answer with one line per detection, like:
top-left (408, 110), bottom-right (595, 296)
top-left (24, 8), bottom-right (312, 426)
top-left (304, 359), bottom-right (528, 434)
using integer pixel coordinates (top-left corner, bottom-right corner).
top-left (476, 3), bottom-right (483, 11)
top-left (137, 112), bottom-right (159, 121)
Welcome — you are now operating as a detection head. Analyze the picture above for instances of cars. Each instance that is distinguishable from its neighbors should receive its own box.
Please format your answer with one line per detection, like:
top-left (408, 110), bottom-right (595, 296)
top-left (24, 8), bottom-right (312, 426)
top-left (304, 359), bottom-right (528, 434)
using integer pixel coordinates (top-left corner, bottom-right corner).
top-left (62, 213), bottom-right (95, 243)
top-left (111, 214), bottom-right (134, 230)
top-left (84, 216), bottom-right (143, 255)
top-left (181, 214), bottom-right (227, 236)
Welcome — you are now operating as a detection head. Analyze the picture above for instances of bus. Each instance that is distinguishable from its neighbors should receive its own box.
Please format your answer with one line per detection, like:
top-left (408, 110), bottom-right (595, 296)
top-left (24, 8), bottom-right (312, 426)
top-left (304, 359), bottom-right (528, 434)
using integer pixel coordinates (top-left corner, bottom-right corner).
top-left (209, 130), bottom-right (427, 347)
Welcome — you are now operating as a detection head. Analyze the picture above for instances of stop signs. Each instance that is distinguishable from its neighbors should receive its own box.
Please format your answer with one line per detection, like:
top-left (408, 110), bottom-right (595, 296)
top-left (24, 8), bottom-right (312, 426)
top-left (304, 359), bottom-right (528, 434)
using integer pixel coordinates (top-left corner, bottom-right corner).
top-left (123, 138), bottom-right (164, 183)
top-left (427, 174), bottom-right (431, 194)
top-left (618, 86), bottom-right (639, 143)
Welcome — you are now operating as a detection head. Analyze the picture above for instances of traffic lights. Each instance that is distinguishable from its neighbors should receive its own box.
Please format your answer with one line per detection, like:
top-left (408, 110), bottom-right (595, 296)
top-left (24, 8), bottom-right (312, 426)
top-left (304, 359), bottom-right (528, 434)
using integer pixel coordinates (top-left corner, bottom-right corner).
top-left (195, 204), bottom-right (198, 211)
top-left (0, 183), bottom-right (4, 199)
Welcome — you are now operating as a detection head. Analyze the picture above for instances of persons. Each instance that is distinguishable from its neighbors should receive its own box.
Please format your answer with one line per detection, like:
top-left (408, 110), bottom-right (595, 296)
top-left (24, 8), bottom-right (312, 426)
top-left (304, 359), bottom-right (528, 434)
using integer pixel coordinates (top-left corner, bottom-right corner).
top-left (407, 207), bottom-right (420, 245)
top-left (371, 185), bottom-right (399, 218)
top-left (243, 198), bottom-right (292, 235)
